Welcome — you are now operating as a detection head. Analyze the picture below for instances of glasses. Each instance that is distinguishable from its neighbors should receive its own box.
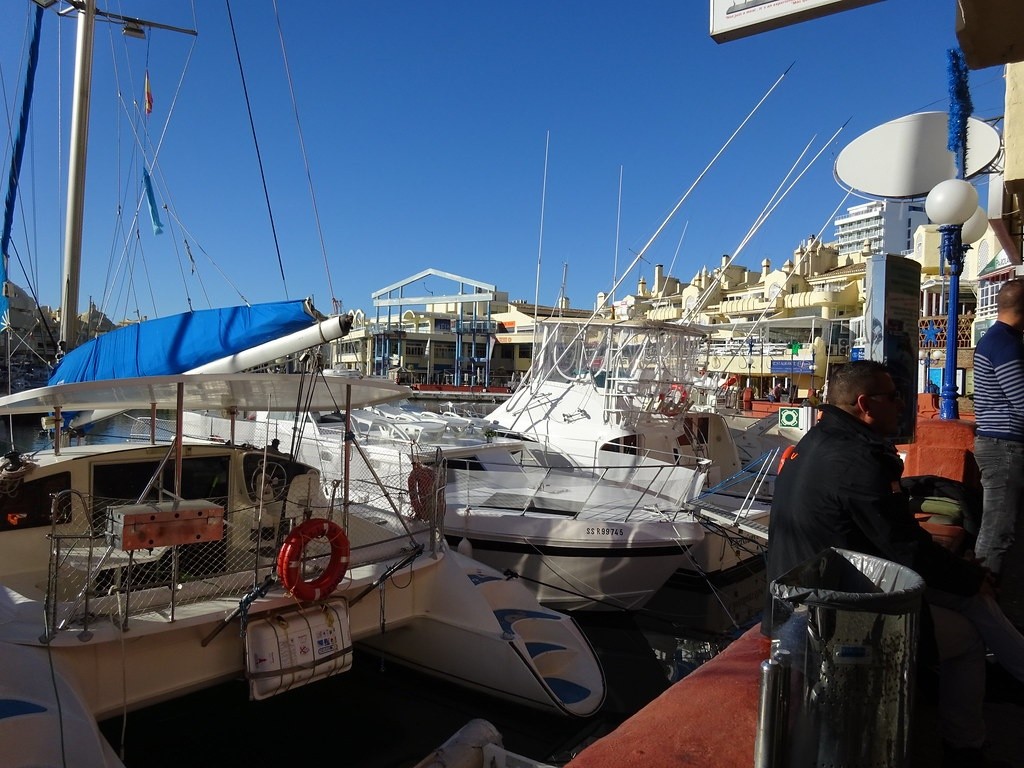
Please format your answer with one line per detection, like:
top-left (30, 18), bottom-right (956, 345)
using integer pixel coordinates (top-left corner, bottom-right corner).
top-left (849, 389), bottom-right (901, 407)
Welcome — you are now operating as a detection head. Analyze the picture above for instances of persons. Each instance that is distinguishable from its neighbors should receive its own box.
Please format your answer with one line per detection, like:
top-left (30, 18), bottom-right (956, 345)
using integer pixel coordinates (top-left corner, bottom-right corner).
top-left (767, 379), bottom-right (821, 407)
top-left (974, 275), bottom-right (1023, 601)
top-left (757, 360), bottom-right (1023, 767)
top-left (924, 379), bottom-right (941, 396)
top-left (263, 437), bottom-right (292, 458)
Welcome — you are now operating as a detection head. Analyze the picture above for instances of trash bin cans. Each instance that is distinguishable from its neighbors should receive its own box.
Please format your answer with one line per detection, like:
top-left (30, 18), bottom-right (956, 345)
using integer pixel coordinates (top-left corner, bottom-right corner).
top-left (769, 542), bottom-right (926, 767)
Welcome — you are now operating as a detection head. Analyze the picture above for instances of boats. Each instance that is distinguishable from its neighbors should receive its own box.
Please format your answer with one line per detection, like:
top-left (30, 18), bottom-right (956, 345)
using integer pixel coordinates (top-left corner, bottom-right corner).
top-left (0, 0), bottom-right (855, 768)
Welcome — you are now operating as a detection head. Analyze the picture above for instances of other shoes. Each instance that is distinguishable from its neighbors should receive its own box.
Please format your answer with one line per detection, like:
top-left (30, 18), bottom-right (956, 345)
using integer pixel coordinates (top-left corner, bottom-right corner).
top-left (940, 740), bottom-right (1011, 767)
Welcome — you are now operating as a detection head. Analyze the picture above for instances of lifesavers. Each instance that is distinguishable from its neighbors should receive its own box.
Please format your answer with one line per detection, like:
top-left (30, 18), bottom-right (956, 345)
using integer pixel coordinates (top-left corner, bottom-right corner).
top-left (776, 444), bottom-right (797, 476)
top-left (276, 518), bottom-right (352, 605)
top-left (408, 466), bottom-right (447, 525)
top-left (657, 383), bottom-right (689, 417)
